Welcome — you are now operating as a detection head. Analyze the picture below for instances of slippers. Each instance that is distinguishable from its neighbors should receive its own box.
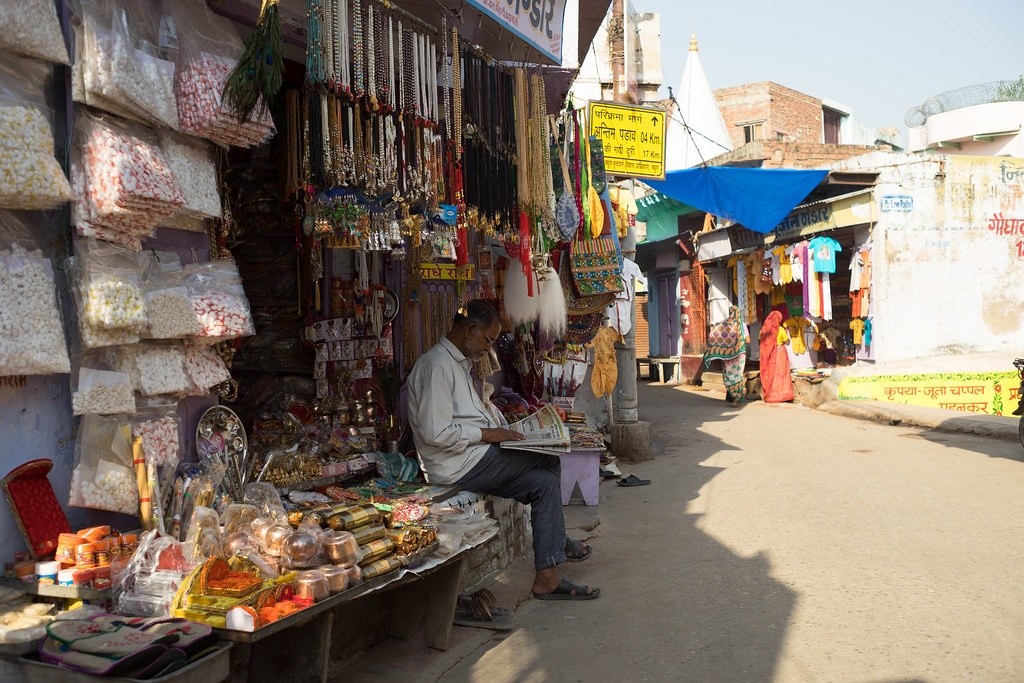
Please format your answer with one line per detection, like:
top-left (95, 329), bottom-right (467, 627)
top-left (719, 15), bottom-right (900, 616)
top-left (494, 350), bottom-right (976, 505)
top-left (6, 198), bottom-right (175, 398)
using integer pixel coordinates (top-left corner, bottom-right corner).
top-left (454, 597), bottom-right (514, 628)
top-left (533, 578), bottom-right (600, 600)
top-left (563, 537), bottom-right (593, 563)
top-left (616, 474), bottom-right (651, 486)
top-left (599, 468), bottom-right (613, 477)
top-left (457, 590), bottom-right (515, 618)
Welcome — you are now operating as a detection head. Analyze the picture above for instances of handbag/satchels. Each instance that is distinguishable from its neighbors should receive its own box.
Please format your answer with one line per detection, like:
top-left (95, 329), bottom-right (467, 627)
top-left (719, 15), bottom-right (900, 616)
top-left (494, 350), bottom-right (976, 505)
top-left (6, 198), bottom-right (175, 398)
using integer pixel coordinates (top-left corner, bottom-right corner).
top-left (548, 107), bottom-right (627, 297)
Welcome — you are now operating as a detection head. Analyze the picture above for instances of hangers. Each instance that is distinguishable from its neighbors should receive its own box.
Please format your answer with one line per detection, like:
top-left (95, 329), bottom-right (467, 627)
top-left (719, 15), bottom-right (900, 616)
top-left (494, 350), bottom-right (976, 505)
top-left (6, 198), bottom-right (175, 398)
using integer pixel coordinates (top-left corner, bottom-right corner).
top-left (738, 232), bottom-right (828, 257)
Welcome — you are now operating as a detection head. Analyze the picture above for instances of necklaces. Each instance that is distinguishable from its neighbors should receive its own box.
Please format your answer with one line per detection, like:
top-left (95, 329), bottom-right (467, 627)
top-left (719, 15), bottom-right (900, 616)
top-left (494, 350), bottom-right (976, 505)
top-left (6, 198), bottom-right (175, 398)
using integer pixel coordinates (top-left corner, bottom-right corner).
top-left (287, 0), bottom-right (555, 379)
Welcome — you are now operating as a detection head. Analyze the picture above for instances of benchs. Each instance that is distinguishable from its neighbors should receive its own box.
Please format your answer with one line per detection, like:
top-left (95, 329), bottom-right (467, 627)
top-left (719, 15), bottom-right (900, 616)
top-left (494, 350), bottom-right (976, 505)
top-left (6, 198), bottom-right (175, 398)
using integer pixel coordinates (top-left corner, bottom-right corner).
top-left (636, 357), bottom-right (680, 386)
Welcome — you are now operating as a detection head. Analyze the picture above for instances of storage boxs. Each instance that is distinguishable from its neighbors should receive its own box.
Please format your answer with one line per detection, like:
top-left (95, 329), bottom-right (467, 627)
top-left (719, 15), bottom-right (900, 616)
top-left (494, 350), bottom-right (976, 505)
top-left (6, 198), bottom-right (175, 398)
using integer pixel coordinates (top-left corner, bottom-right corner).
top-left (547, 395), bottom-right (576, 412)
top-left (0, 585), bottom-right (233, 683)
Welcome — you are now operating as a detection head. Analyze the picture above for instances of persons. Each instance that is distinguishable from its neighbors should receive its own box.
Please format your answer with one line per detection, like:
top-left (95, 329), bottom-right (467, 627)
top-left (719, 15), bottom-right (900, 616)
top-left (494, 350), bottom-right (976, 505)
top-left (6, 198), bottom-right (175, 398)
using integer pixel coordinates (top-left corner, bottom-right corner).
top-left (406, 299), bottom-right (600, 601)
top-left (759, 311), bottom-right (795, 403)
top-left (704, 306), bottom-right (750, 408)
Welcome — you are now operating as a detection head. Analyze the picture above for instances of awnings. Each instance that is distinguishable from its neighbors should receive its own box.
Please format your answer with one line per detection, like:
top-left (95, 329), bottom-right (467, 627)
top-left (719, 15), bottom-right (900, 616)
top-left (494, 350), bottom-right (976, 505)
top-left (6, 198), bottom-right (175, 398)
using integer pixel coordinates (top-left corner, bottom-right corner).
top-left (697, 186), bottom-right (874, 238)
top-left (636, 229), bottom-right (697, 263)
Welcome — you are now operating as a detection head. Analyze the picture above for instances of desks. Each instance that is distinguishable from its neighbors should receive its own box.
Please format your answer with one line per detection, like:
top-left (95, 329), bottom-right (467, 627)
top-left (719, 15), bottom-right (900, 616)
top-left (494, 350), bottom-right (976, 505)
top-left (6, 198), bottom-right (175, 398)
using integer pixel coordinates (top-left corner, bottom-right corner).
top-left (117, 463), bottom-right (501, 683)
top-left (556, 423), bottom-right (606, 506)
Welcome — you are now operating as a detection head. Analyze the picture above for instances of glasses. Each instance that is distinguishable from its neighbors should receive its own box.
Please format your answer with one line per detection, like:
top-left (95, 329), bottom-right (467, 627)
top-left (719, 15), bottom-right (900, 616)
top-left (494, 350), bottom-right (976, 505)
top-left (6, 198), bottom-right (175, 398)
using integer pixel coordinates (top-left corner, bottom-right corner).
top-left (467, 321), bottom-right (494, 347)
